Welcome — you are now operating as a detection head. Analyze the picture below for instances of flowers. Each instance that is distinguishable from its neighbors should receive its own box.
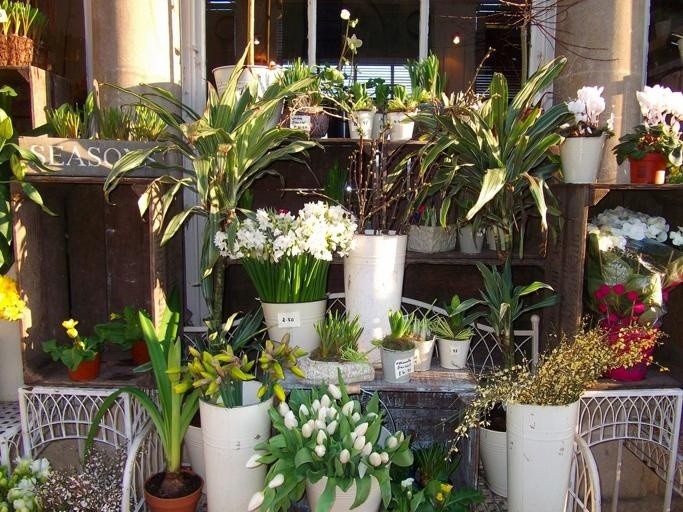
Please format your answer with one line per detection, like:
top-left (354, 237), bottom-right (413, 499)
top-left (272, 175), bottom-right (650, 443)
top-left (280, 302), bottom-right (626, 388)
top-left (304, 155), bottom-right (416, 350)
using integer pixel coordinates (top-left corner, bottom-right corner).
top-left (0, 9), bottom-right (682, 512)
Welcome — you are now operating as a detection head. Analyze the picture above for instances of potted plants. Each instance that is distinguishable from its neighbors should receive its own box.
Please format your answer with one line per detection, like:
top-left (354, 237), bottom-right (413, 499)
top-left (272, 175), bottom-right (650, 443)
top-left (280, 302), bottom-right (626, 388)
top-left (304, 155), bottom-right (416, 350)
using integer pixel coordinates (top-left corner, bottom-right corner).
top-left (0, 0), bottom-right (38, 69)
